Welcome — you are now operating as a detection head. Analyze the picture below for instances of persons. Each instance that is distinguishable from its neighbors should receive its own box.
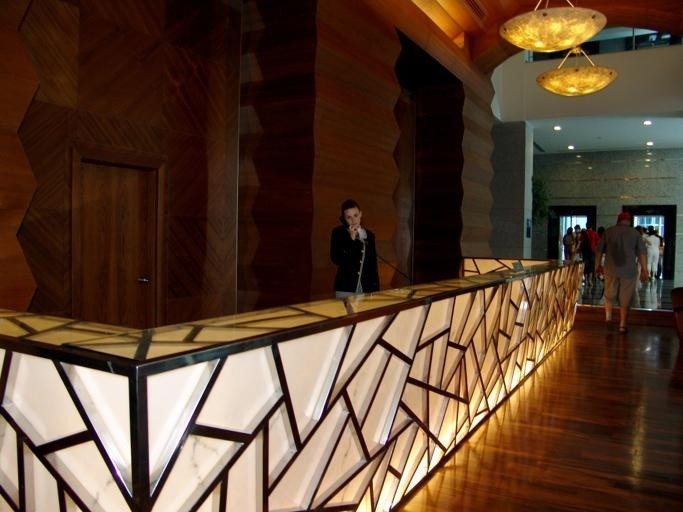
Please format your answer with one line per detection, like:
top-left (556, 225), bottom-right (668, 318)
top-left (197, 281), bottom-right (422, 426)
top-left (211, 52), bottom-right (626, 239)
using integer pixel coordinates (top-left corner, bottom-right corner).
top-left (562, 212), bottom-right (665, 335)
top-left (330, 199), bottom-right (380, 299)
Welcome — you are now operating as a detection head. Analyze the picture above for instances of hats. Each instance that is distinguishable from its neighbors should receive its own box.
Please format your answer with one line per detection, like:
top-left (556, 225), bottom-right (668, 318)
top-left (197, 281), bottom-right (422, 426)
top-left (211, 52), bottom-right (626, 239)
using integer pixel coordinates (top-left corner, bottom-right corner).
top-left (617, 212), bottom-right (631, 221)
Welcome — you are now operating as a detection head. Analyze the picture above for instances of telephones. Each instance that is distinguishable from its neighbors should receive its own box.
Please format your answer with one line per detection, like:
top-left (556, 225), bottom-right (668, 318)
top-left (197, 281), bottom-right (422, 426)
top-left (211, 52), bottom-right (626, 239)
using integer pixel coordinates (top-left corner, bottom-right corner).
top-left (340, 216), bottom-right (359, 238)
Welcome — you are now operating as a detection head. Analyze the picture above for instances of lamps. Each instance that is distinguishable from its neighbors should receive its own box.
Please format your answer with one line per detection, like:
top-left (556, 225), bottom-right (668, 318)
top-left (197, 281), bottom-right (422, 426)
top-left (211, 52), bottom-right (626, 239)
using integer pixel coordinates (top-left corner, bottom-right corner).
top-left (499, 0), bottom-right (608, 53)
top-left (536, 47), bottom-right (618, 97)
